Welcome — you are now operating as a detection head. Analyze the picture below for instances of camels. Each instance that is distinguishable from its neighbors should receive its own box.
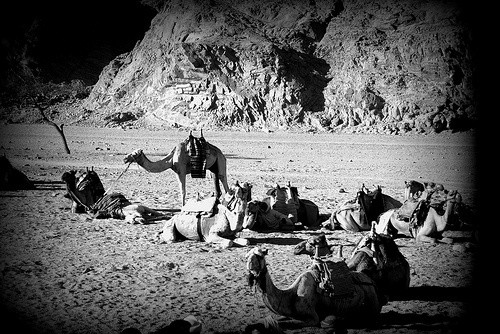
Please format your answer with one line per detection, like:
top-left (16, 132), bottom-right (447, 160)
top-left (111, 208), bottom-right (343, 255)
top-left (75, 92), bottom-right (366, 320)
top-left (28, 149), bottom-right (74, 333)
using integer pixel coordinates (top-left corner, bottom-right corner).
top-left (374, 179), bottom-right (463, 244)
top-left (290, 221), bottom-right (412, 304)
top-left (93, 193), bottom-right (170, 226)
top-left (158, 181), bottom-right (320, 248)
top-left (63, 165), bottom-right (107, 214)
top-left (320, 183), bottom-right (404, 233)
top-left (121, 128), bottom-right (231, 207)
top-left (245, 248), bottom-right (380, 334)
